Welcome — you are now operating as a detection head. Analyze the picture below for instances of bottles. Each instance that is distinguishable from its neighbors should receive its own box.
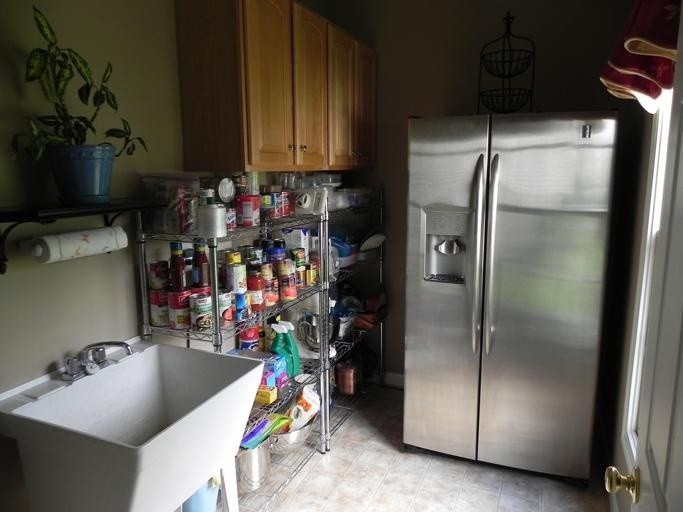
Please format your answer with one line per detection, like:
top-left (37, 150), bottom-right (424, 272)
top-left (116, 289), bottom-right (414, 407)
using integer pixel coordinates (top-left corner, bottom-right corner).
top-left (170, 241), bottom-right (187, 292)
top-left (192, 238), bottom-right (211, 287)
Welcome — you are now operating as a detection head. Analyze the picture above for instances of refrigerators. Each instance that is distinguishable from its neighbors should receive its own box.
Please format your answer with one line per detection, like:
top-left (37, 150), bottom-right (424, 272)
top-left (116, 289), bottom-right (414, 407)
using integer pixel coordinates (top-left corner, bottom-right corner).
top-left (403, 110), bottom-right (619, 481)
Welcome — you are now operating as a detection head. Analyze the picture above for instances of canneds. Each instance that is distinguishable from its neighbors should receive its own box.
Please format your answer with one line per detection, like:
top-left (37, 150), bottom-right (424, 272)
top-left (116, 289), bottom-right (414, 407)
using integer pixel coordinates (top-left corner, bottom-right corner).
top-left (145, 173), bottom-right (320, 331)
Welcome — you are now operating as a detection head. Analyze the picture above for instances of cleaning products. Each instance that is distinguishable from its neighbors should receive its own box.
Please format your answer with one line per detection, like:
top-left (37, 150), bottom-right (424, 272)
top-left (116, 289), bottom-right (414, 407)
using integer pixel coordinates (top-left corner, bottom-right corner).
top-left (270, 320), bottom-right (301, 379)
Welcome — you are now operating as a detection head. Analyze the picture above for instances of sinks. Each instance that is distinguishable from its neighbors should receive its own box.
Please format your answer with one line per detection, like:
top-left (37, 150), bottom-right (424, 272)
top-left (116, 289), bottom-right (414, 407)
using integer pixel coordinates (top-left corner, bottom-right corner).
top-left (0, 333), bottom-right (266, 512)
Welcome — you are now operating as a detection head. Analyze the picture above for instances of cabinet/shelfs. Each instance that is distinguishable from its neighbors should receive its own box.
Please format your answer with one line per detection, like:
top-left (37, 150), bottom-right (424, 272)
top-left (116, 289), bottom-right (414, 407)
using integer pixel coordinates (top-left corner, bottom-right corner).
top-left (134, 185), bottom-right (325, 512)
top-left (174, 0), bottom-right (326, 173)
top-left (327, 20), bottom-right (378, 171)
top-left (324, 186), bottom-right (386, 450)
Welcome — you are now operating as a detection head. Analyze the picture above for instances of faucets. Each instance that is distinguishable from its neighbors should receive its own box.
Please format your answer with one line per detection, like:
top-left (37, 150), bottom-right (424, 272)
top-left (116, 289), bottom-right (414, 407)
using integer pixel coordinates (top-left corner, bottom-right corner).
top-left (59, 338), bottom-right (134, 379)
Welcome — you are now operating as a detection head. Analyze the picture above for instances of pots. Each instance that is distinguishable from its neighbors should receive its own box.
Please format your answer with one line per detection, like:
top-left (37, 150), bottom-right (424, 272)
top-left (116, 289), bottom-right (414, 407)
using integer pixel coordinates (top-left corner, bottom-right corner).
top-left (235, 415), bottom-right (318, 492)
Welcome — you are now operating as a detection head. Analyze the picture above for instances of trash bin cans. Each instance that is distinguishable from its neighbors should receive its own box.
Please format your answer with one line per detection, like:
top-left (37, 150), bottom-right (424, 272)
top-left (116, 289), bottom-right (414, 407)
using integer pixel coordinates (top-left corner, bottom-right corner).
top-left (182, 470), bottom-right (221, 512)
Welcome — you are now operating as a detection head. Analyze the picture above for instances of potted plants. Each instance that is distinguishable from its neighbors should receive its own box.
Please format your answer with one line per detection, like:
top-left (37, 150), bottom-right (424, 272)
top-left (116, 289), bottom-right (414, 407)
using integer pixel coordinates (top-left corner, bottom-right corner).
top-left (8, 4), bottom-right (149, 210)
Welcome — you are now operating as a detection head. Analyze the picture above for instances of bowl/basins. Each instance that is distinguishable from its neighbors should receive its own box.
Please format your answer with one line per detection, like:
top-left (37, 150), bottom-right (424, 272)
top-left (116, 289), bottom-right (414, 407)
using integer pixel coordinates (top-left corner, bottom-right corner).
top-left (298, 316), bottom-right (340, 352)
